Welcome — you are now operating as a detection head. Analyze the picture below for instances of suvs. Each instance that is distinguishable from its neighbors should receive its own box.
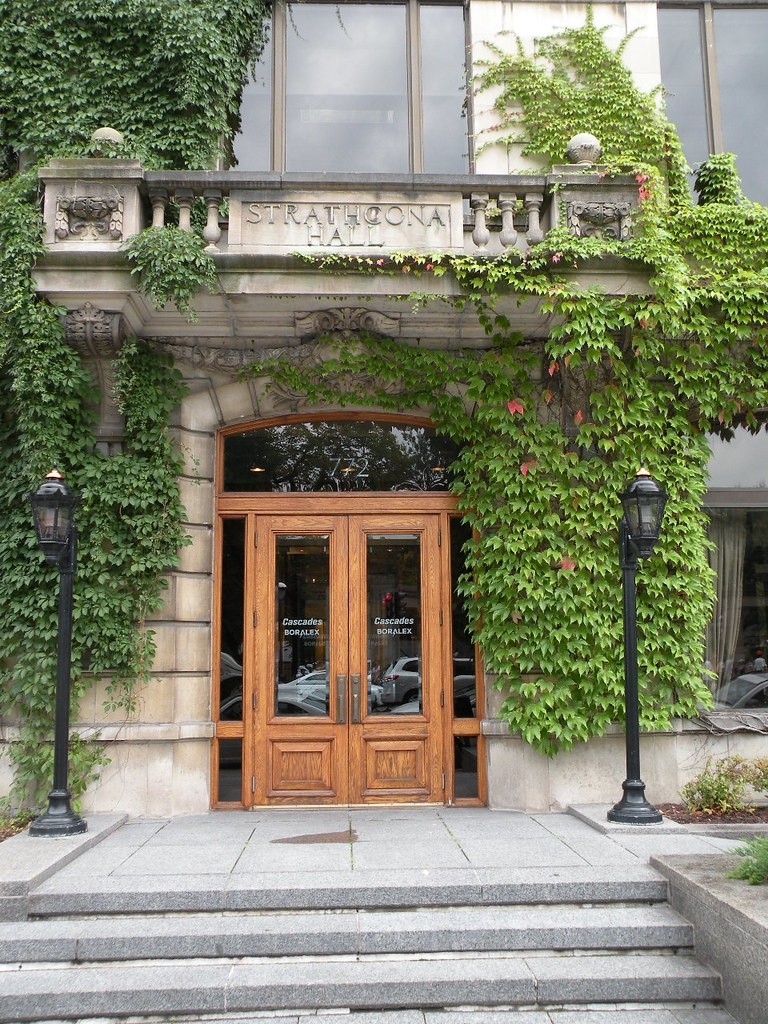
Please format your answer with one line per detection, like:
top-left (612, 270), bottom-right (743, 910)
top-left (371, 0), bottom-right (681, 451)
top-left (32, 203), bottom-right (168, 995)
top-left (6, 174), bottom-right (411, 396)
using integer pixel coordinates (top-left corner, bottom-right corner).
top-left (380, 656), bottom-right (475, 704)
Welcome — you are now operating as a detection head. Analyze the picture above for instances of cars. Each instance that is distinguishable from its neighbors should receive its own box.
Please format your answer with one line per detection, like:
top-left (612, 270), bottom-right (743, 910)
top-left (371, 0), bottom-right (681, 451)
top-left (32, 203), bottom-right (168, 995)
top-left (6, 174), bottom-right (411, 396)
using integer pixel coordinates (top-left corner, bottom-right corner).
top-left (278, 670), bottom-right (385, 710)
top-left (218, 652), bottom-right (332, 767)
top-left (704, 673), bottom-right (768, 710)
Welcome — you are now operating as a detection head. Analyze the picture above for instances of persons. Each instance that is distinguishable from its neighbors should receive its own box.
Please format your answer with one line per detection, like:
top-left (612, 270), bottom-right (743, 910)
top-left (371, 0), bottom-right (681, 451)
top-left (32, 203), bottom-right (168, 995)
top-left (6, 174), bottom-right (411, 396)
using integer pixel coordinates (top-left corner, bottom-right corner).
top-left (702, 650), bottom-right (768, 693)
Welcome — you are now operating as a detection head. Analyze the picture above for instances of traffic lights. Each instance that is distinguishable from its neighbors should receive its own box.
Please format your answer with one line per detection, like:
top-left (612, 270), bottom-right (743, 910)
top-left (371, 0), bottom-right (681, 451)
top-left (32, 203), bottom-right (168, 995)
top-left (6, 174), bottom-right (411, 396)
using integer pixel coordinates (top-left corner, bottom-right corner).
top-left (382, 592), bottom-right (393, 619)
top-left (396, 592), bottom-right (407, 618)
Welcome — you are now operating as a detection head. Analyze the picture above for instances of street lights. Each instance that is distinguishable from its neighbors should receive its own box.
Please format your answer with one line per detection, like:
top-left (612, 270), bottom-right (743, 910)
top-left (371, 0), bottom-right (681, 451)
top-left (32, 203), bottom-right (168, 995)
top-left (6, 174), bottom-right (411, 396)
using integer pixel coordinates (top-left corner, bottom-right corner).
top-left (278, 581), bottom-right (287, 679)
top-left (608, 468), bottom-right (662, 824)
top-left (30, 466), bottom-right (87, 837)
